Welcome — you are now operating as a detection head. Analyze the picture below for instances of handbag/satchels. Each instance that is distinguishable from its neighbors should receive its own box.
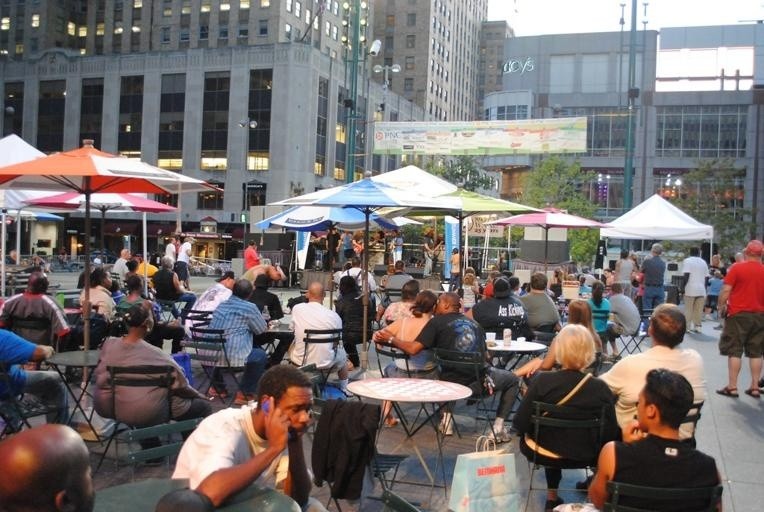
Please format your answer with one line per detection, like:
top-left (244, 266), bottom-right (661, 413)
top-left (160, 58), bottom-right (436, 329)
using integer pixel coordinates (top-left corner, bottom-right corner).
top-left (447, 436), bottom-right (520, 512)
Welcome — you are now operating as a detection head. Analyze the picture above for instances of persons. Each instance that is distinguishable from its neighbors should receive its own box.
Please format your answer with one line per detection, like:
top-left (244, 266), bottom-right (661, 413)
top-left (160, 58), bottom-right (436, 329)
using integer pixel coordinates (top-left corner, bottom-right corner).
top-left (0, 228), bottom-right (764, 511)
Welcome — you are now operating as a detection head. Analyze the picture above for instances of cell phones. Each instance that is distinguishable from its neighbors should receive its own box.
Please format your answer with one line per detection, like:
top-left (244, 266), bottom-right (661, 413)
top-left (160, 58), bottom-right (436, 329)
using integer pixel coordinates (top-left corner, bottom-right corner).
top-left (260, 397), bottom-right (295, 442)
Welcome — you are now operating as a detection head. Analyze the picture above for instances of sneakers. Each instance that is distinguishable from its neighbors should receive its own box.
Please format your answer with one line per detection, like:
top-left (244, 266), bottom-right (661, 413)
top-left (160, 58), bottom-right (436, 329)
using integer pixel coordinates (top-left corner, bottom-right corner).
top-left (487, 426), bottom-right (511, 444)
top-left (208, 387), bottom-right (227, 398)
top-left (545, 496), bottom-right (564, 512)
top-left (576, 475), bottom-right (593, 489)
top-left (235, 391), bottom-right (258, 405)
top-left (438, 420), bottom-right (454, 435)
top-left (692, 325), bottom-right (702, 332)
top-left (384, 414), bottom-right (401, 427)
top-left (601, 352), bottom-right (622, 363)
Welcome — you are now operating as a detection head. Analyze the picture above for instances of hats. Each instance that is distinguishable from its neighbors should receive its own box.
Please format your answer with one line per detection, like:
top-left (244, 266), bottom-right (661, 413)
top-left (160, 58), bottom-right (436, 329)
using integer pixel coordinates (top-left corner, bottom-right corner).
top-left (745, 240), bottom-right (764, 256)
top-left (493, 276), bottom-right (513, 298)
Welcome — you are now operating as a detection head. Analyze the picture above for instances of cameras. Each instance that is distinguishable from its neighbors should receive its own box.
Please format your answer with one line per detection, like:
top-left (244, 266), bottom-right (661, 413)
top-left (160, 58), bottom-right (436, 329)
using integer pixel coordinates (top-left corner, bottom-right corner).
top-left (288, 296), bottom-right (307, 308)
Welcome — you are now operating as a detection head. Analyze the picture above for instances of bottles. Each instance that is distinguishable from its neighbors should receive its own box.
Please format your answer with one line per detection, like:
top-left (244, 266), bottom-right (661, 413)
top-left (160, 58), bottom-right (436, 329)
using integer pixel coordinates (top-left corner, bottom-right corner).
top-left (261, 305), bottom-right (270, 330)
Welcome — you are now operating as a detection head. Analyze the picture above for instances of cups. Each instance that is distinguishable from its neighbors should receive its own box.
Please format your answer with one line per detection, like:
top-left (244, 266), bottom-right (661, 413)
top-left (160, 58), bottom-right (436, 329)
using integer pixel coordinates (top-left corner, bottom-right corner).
top-left (517, 337), bottom-right (526, 348)
top-left (486, 332), bottom-right (496, 347)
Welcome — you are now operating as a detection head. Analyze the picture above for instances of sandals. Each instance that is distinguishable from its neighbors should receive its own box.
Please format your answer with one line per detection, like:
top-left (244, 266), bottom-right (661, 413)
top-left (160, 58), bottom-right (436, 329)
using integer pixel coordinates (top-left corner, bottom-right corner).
top-left (745, 388), bottom-right (760, 397)
top-left (716, 386), bottom-right (738, 397)
